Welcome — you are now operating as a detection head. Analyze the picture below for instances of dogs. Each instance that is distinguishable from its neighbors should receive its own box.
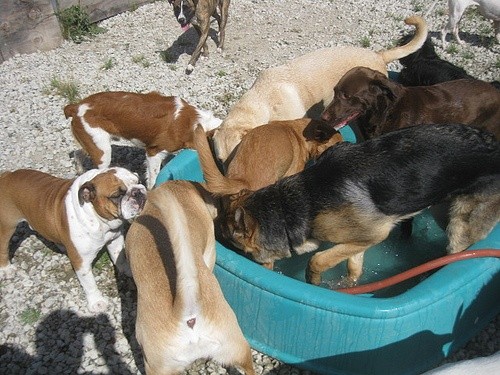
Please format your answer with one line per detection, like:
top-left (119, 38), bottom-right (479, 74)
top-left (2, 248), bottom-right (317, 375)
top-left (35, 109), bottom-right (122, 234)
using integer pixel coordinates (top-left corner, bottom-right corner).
top-left (211, 15), bottom-right (428, 177)
top-left (64, 88), bottom-right (223, 192)
top-left (319, 67), bottom-right (500, 143)
top-left (124, 181), bottom-right (258, 375)
top-left (168, 0), bottom-right (231, 75)
top-left (192, 117), bottom-right (343, 198)
top-left (0, 166), bottom-right (149, 313)
top-left (440, 0), bottom-right (500, 50)
top-left (215, 122), bottom-right (500, 289)
